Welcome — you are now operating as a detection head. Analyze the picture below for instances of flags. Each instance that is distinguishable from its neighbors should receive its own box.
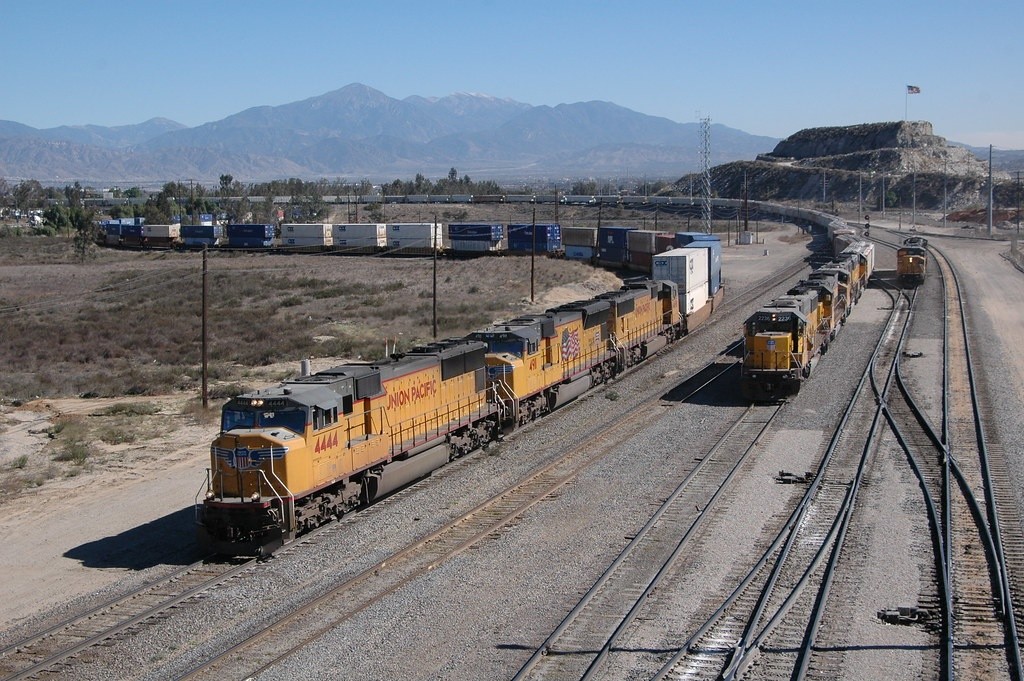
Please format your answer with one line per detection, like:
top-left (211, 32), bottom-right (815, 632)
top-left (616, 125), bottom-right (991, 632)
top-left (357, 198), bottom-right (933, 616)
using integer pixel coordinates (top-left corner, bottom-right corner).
top-left (907, 86), bottom-right (921, 94)
top-left (561, 327), bottom-right (581, 361)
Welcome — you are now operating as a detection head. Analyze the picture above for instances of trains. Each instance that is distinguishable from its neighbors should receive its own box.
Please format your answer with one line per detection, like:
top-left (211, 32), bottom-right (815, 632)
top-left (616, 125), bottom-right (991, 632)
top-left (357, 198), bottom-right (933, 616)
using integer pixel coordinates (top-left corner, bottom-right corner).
top-left (99, 217), bottom-right (726, 563)
top-left (47, 193), bottom-right (877, 403)
top-left (896, 236), bottom-right (929, 287)
